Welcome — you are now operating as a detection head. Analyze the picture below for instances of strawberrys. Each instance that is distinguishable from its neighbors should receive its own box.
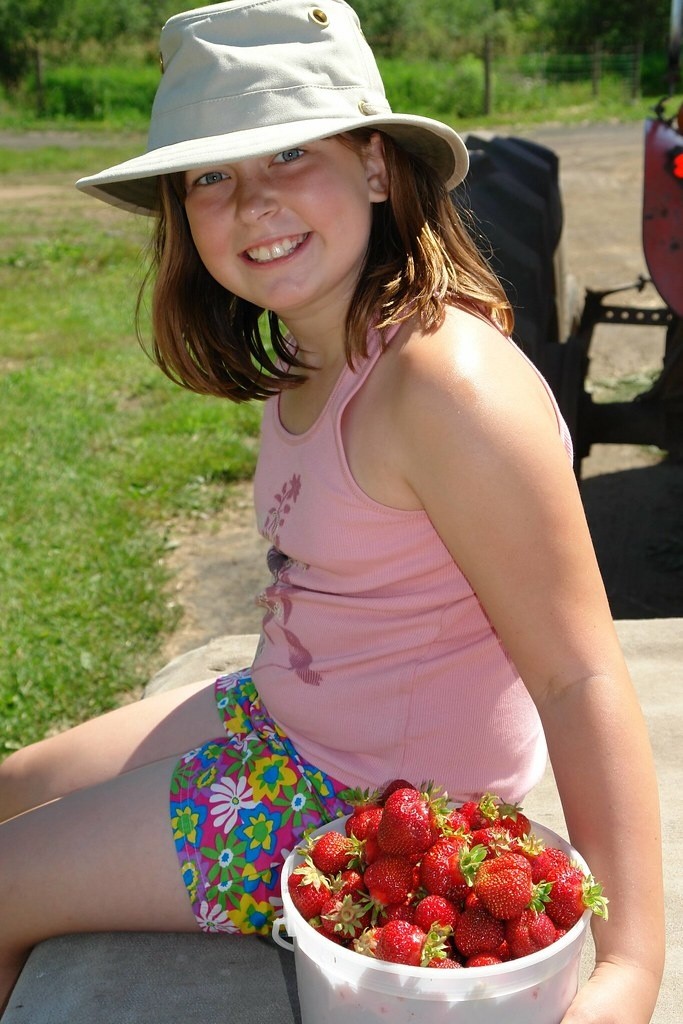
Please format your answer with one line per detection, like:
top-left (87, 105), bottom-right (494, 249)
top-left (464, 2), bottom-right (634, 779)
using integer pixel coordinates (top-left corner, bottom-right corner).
top-left (287, 778), bottom-right (609, 968)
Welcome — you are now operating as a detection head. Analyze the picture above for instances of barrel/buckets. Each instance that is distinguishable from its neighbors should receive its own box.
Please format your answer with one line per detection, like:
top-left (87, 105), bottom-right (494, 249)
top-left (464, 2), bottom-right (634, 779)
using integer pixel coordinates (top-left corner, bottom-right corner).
top-left (270, 796), bottom-right (597, 1024)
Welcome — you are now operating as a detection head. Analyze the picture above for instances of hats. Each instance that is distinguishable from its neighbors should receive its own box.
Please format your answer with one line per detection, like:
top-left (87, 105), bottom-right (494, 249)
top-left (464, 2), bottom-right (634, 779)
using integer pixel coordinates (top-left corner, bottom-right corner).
top-left (75, 1), bottom-right (469, 218)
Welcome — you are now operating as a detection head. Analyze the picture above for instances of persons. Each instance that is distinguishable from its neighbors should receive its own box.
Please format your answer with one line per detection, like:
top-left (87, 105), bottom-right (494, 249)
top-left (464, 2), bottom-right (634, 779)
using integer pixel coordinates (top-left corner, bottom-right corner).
top-left (0, 1), bottom-right (666, 1024)
top-left (634, 100), bottom-right (683, 428)
